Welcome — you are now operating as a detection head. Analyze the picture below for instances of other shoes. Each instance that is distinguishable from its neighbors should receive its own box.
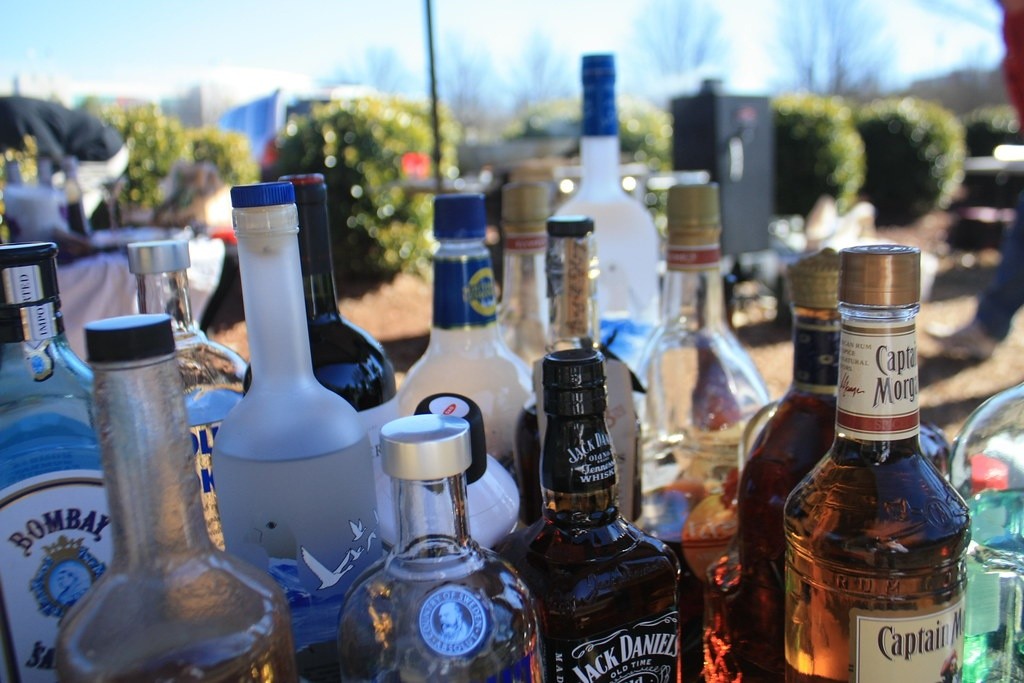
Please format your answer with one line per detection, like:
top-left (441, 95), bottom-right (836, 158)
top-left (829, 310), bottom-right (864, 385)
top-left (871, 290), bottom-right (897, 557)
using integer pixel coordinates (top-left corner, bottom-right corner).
top-left (924, 321), bottom-right (995, 360)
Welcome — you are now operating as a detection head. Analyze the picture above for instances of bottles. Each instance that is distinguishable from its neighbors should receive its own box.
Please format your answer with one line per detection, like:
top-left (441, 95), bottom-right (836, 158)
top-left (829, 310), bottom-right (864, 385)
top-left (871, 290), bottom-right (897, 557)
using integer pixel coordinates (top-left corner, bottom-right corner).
top-left (490, 350), bottom-right (681, 683)
top-left (374, 393), bottom-right (519, 556)
top-left (337, 414), bottom-right (544, 683)
top-left (56, 314), bottom-right (299, 683)
top-left (635, 185), bottom-right (770, 542)
top-left (549, 55), bottom-right (660, 409)
top-left (398, 196), bottom-right (532, 489)
top-left (532, 219), bottom-right (640, 524)
top-left (0, 243), bottom-right (113, 683)
top-left (212, 183), bottom-right (383, 652)
top-left (495, 181), bottom-right (551, 384)
top-left (784, 244), bottom-right (971, 683)
top-left (703, 248), bottom-right (842, 683)
top-left (244, 174), bottom-right (396, 486)
top-left (101, 240), bottom-right (248, 554)
top-left (950, 380), bottom-right (1024, 683)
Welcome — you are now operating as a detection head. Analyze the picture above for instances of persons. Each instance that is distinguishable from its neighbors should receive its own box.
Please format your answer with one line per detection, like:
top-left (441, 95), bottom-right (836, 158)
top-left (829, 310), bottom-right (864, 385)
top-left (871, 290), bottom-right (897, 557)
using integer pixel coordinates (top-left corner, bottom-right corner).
top-left (923, 1), bottom-right (1024, 358)
top-left (0, 98), bottom-right (129, 220)
